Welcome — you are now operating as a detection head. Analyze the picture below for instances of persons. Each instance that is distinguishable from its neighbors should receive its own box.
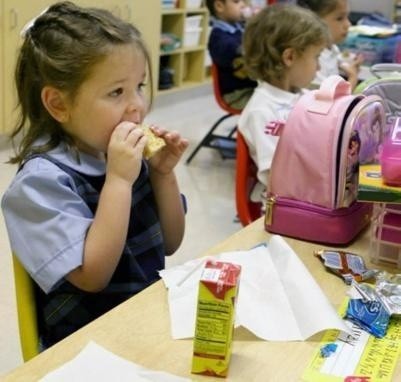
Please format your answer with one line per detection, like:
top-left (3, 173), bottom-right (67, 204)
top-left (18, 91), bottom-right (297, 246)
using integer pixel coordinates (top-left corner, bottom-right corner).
top-left (1, 1), bottom-right (189, 355)
top-left (294, 0), bottom-right (365, 92)
top-left (204, 0), bottom-right (259, 112)
top-left (237, 3), bottom-right (333, 217)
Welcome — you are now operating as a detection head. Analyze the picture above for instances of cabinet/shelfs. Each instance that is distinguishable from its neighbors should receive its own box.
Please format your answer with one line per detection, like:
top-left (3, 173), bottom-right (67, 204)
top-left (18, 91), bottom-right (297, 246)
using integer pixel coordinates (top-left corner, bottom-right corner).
top-left (160, 1), bottom-right (211, 94)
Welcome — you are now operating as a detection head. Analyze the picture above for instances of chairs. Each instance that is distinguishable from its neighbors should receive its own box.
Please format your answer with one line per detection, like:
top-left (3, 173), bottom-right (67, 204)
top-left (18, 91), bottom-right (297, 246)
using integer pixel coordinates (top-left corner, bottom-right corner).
top-left (184, 63), bottom-right (261, 227)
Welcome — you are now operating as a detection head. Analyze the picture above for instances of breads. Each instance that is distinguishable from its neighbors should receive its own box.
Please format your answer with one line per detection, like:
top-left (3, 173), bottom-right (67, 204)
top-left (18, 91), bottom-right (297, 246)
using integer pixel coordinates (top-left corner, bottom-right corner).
top-left (138, 122), bottom-right (166, 161)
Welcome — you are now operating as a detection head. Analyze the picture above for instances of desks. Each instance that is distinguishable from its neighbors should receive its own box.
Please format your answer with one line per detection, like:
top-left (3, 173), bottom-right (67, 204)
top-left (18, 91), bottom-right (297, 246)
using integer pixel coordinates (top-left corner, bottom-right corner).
top-left (2, 209), bottom-right (400, 381)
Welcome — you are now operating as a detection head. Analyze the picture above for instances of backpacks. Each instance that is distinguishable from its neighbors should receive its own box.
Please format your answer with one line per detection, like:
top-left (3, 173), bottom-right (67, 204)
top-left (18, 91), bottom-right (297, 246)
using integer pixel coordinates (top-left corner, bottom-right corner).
top-left (264, 74), bottom-right (386, 246)
